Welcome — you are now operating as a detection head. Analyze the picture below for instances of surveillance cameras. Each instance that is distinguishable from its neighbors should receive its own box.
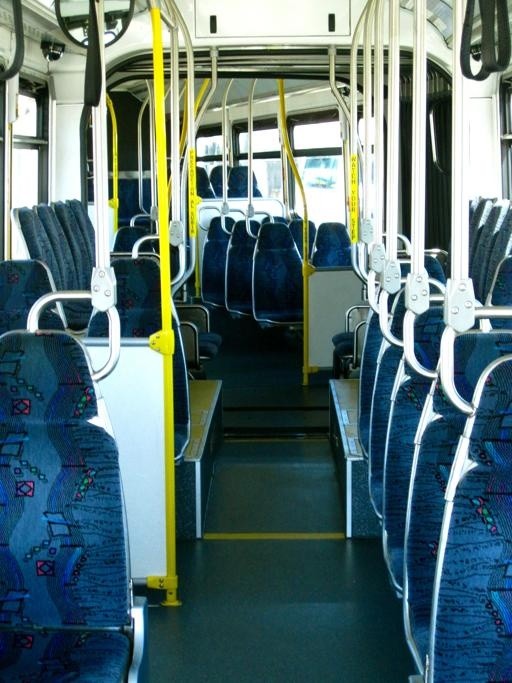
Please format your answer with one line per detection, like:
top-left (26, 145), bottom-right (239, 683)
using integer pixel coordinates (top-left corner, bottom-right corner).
top-left (40, 40), bottom-right (65, 62)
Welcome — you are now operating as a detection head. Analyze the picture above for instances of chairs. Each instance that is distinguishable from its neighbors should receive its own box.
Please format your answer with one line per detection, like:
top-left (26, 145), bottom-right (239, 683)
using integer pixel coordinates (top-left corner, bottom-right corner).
top-left (1, 165), bottom-right (511, 682)
